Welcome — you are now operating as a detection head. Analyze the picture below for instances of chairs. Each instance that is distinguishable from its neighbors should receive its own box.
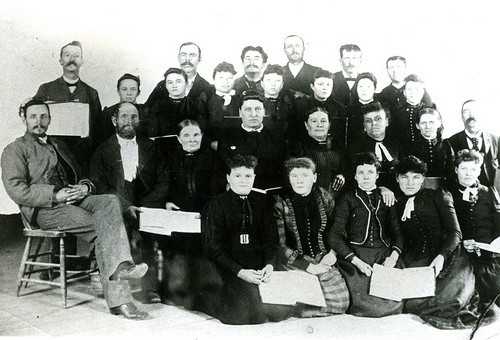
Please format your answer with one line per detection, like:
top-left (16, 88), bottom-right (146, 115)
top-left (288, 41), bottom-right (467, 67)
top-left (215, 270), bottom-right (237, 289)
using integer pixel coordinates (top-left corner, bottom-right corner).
top-left (16, 204), bottom-right (100, 309)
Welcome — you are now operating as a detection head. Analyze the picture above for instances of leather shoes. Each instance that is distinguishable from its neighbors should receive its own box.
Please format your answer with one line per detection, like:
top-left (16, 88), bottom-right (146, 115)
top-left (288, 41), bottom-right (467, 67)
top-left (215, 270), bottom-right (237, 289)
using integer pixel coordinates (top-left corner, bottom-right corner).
top-left (109, 262), bottom-right (149, 282)
top-left (109, 302), bottom-right (152, 320)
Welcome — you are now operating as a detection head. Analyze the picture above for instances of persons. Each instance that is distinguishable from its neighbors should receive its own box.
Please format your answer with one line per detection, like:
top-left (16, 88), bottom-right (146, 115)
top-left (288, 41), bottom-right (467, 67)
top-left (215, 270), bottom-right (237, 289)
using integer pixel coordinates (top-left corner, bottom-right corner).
top-left (378, 155), bottom-right (495, 330)
top-left (20, 41), bottom-right (101, 281)
top-left (328, 154), bottom-right (405, 317)
top-left (0, 101), bottom-right (150, 319)
top-left (90, 103), bottom-right (163, 302)
top-left (148, 35), bottom-right (500, 210)
top-left (186, 156), bottom-right (290, 324)
top-left (274, 158), bottom-right (349, 317)
top-left (441, 149), bottom-right (500, 308)
top-left (102, 74), bottom-right (141, 139)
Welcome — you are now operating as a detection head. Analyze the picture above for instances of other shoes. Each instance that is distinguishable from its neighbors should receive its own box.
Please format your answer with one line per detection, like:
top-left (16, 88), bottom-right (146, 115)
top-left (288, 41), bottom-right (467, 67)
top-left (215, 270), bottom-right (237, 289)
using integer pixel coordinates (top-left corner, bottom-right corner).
top-left (148, 292), bottom-right (175, 306)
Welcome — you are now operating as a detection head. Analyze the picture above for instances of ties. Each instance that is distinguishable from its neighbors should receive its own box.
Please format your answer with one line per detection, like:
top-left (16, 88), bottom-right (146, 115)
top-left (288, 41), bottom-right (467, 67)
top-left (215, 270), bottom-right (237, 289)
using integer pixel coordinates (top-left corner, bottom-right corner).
top-left (470, 138), bottom-right (479, 151)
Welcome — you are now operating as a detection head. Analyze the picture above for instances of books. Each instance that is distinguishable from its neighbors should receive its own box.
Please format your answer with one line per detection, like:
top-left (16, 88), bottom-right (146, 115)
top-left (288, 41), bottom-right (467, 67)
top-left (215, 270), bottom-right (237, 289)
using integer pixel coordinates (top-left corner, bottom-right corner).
top-left (138, 207), bottom-right (202, 237)
top-left (257, 269), bottom-right (326, 308)
top-left (475, 236), bottom-right (500, 254)
top-left (369, 263), bottom-right (435, 302)
